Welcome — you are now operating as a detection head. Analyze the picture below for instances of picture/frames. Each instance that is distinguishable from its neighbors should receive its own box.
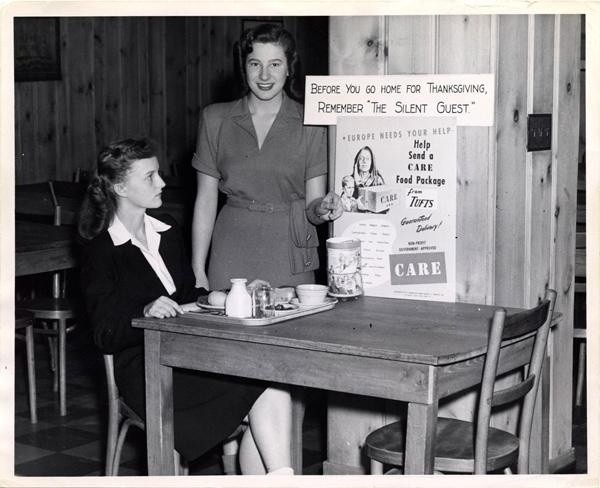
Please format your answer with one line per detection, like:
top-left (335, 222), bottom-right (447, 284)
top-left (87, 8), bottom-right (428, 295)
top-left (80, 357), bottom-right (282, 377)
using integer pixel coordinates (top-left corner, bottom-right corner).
top-left (14, 17), bottom-right (61, 83)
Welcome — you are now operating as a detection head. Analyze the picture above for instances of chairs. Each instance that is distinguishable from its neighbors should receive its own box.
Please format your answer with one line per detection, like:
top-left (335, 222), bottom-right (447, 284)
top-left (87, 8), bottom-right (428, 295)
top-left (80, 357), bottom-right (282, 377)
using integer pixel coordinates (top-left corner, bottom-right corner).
top-left (361, 288), bottom-right (557, 475)
top-left (101, 354), bottom-right (190, 476)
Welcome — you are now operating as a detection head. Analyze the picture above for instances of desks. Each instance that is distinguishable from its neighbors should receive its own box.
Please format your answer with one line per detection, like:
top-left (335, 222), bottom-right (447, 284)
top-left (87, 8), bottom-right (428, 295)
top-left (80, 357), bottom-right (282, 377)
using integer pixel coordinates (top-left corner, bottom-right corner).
top-left (132, 283), bottom-right (557, 475)
top-left (15, 211), bottom-right (85, 371)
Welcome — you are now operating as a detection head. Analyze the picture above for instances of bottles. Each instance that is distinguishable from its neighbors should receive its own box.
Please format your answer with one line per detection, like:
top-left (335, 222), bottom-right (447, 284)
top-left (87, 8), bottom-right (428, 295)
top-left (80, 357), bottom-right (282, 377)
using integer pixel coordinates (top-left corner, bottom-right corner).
top-left (226, 278), bottom-right (255, 318)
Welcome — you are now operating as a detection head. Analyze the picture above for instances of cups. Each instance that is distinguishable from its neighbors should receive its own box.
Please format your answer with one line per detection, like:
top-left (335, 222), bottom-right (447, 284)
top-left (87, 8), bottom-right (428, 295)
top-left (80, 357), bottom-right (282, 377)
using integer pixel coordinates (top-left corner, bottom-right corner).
top-left (297, 284), bottom-right (330, 304)
top-left (207, 292), bottom-right (226, 306)
top-left (326, 237), bottom-right (362, 299)
top-left (252, 282), bottom-right (276, 318)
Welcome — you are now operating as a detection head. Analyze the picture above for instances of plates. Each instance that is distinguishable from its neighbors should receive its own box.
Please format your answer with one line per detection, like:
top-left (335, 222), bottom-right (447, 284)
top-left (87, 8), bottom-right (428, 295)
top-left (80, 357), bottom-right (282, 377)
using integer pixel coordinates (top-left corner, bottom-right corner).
top-left (196, 294), bottom-right (227, 310)
top-left (289, 296), bottom-right (339, 308)
top-left (261, 302), bottom-right (299, 316)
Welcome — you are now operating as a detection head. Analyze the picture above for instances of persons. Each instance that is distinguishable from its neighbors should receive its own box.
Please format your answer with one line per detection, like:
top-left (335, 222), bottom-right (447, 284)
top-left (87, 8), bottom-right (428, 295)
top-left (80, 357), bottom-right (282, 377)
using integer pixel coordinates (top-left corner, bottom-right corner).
top-left (76, 135), bottom-right (295, 476)
top-left (349, 146), bottom-right (390, 212)
top-left (336, 175), bottom-right (360, 213)
top-left (191, 25), bottom-right (343, 476)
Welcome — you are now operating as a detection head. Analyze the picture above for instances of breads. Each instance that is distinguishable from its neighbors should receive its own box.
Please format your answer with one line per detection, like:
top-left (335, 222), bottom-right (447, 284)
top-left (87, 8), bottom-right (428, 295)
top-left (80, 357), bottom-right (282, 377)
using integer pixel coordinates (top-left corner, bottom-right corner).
top-left (207, 290), bottom-right (226, 307)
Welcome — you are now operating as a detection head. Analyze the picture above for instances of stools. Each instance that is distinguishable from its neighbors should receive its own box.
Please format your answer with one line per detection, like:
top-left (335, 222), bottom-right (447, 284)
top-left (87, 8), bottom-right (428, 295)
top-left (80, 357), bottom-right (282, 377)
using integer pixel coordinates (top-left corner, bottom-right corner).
top-left (15, 310), bottom-right (35, 423)
top-left (28, 298), bottom-right (83, 415)
